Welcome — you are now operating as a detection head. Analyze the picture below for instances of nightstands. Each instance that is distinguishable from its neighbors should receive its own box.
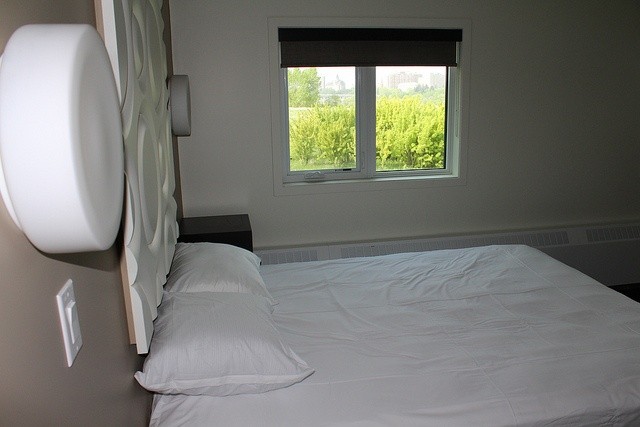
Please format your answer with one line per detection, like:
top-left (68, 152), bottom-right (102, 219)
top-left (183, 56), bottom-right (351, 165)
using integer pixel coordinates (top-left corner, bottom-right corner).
top-left (177, 213), bottom-right (254, 252)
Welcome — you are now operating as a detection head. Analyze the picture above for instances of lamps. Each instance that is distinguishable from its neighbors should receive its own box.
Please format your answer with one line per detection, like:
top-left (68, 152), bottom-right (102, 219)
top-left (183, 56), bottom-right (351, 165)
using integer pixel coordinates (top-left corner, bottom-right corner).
top-left (1, 20), bottom-right (126, 256)
top-left (169, 75), bottom-right (193, 138)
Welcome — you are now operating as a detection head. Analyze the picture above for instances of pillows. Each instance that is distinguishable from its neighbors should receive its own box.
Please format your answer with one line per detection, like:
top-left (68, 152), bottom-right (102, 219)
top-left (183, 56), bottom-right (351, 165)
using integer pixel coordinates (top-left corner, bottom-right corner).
top-left (135, 294), bottom-right (316, 394)
top-left (161, 242), bottom-right (278, 305)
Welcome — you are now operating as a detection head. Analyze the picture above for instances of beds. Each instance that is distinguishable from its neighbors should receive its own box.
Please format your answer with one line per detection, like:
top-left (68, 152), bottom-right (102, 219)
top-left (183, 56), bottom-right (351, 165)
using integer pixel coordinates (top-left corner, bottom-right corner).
top-left (148, 242), bottom-right (640, 426)
